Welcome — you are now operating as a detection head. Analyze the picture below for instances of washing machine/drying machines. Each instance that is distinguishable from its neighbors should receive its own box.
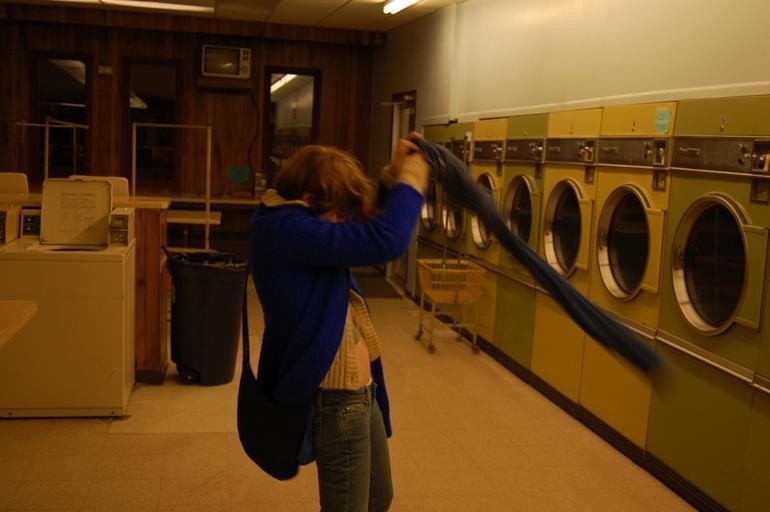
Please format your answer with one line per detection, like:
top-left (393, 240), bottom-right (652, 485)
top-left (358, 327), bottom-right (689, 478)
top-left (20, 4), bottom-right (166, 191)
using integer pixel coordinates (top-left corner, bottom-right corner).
top-left (412, 85), bottom-right (768, 512)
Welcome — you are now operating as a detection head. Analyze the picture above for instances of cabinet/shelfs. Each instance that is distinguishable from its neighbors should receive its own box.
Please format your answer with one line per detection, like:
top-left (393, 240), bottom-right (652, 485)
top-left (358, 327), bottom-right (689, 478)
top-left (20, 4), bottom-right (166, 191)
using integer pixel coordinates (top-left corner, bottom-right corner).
top-left (176, 69), bottom-right (266, 201)
top-left (112, 192), bottom-right (171, 385)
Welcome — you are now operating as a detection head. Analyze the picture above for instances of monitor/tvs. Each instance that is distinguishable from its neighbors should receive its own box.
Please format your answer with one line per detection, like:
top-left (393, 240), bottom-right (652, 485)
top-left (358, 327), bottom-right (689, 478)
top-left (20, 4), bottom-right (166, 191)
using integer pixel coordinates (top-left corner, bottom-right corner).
top-left (199, 43), bottom-right (251, 80)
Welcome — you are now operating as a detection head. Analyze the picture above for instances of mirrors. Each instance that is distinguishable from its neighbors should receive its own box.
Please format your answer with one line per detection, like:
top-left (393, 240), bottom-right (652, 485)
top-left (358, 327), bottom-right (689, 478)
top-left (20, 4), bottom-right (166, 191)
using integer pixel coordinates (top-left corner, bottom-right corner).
top-left (260, 63), bottom-right (325, 182)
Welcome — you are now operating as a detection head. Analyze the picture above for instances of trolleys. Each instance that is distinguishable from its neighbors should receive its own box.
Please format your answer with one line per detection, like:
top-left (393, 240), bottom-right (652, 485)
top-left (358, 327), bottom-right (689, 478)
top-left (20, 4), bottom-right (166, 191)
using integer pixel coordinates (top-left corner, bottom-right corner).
top-left (416, 257), bottom-right (487, 355)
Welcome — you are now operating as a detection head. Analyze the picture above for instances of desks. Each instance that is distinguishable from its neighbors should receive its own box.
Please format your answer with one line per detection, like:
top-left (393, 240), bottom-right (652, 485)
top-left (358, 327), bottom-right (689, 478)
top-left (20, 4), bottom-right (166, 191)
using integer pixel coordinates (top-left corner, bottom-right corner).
top-left (165, 209), bottom-right (223, 251)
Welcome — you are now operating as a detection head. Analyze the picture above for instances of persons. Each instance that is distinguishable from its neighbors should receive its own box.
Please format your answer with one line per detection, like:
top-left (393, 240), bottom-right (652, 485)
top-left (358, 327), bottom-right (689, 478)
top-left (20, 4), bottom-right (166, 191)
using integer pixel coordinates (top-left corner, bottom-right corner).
top-left (237, 132), bottom-right (430, 512)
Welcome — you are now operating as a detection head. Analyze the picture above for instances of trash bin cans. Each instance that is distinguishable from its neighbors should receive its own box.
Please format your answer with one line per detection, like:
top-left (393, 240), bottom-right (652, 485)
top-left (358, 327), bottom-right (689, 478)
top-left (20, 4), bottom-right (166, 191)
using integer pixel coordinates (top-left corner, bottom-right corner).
top-left (165, 252), bottom-right (248, 386)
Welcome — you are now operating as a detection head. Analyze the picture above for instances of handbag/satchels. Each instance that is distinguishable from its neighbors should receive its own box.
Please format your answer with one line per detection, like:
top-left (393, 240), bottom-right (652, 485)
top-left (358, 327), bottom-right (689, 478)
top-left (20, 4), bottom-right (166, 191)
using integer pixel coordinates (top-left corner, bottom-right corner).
top-left (237, 364), bottom-right (308, 475)
